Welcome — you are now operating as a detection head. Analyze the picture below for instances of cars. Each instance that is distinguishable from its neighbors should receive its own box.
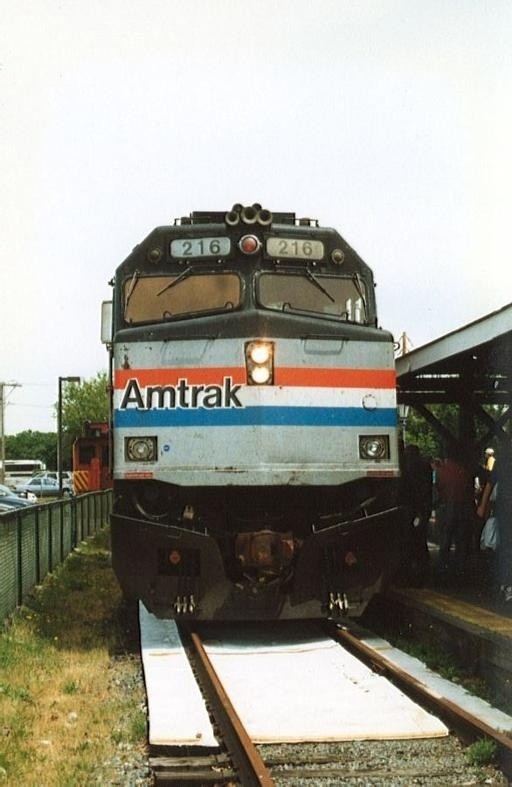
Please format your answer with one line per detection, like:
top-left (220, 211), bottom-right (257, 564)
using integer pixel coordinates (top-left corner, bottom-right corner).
top-left (0, 470), bottom-right (73, 513)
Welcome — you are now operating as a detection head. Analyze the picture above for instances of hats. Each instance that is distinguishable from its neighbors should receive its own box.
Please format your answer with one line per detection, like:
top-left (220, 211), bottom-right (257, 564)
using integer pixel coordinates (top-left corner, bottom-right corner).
top-left (484, 448), bottom-right (495, 455)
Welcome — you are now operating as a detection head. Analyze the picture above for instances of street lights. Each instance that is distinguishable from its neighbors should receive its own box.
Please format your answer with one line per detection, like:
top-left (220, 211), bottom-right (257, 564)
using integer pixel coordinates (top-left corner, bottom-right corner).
top-left (58, 375), bottom-right (80, 497)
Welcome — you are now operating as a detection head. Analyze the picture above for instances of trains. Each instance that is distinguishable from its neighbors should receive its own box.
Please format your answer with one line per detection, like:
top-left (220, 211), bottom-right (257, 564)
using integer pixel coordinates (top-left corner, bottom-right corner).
top-left (101, 202), bottom-right (412, 629)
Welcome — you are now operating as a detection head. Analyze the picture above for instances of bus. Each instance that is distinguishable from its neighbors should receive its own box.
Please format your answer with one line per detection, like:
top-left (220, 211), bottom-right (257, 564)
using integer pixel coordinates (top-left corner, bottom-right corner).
top-left (1, 459), bottom-right (47, 474)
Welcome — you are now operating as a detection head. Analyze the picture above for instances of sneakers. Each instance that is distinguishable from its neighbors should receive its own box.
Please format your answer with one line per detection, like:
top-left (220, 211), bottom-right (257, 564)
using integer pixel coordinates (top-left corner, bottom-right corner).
top-left (502, 584), bottom-right (512, 603)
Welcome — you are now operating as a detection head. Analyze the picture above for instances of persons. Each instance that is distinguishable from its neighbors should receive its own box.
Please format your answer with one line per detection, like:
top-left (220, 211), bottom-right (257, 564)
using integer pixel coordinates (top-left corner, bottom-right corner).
top-left (402, 431), bottom-right (512, 603)
